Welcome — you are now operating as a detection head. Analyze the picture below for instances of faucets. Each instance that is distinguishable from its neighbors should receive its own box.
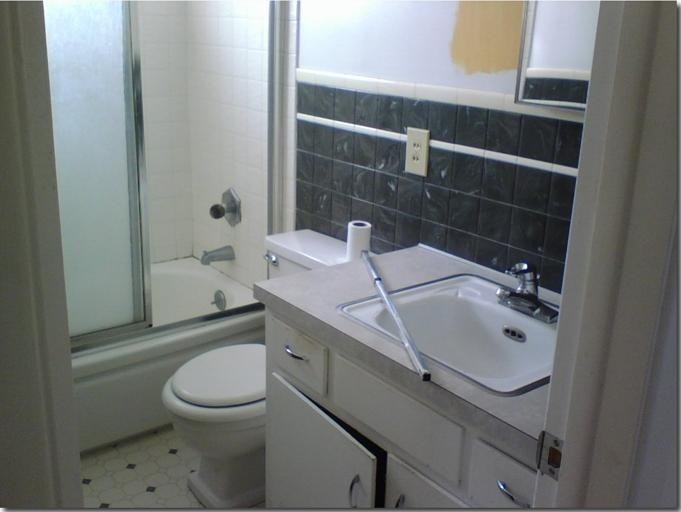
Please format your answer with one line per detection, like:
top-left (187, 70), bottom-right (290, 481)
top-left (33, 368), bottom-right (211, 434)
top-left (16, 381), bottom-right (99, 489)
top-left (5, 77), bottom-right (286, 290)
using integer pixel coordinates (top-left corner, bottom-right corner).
top-left (200, 245), bottom-right (235, 266)
top-left (496, 262), bottom-right (541, 311)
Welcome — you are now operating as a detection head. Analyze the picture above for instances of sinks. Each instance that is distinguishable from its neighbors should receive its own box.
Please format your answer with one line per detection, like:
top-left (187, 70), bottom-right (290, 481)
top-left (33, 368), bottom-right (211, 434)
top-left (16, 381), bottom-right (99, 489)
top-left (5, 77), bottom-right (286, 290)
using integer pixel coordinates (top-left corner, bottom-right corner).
top-left (335, 273), bottom-right (560, 396)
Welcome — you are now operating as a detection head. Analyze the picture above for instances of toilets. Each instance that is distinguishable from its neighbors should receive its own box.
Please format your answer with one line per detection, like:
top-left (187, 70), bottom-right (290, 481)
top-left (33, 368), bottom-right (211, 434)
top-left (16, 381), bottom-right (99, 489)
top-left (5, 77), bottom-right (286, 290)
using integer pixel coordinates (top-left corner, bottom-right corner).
top-left (160, 228), bottom-right (381, 510)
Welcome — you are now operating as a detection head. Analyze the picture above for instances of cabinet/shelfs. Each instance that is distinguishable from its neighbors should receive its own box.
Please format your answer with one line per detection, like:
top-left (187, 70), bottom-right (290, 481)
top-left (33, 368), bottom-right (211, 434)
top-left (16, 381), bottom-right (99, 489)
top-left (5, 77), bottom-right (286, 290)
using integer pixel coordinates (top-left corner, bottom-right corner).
top-left (263, 305), bottom-right (600, 509)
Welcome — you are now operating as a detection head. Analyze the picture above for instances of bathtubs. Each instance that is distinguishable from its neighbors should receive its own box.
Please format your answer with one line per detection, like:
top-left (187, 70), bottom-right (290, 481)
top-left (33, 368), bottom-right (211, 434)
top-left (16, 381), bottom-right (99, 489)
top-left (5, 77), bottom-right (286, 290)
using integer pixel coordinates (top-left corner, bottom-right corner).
top-left (64, 256), bottom-right (265, 453)
